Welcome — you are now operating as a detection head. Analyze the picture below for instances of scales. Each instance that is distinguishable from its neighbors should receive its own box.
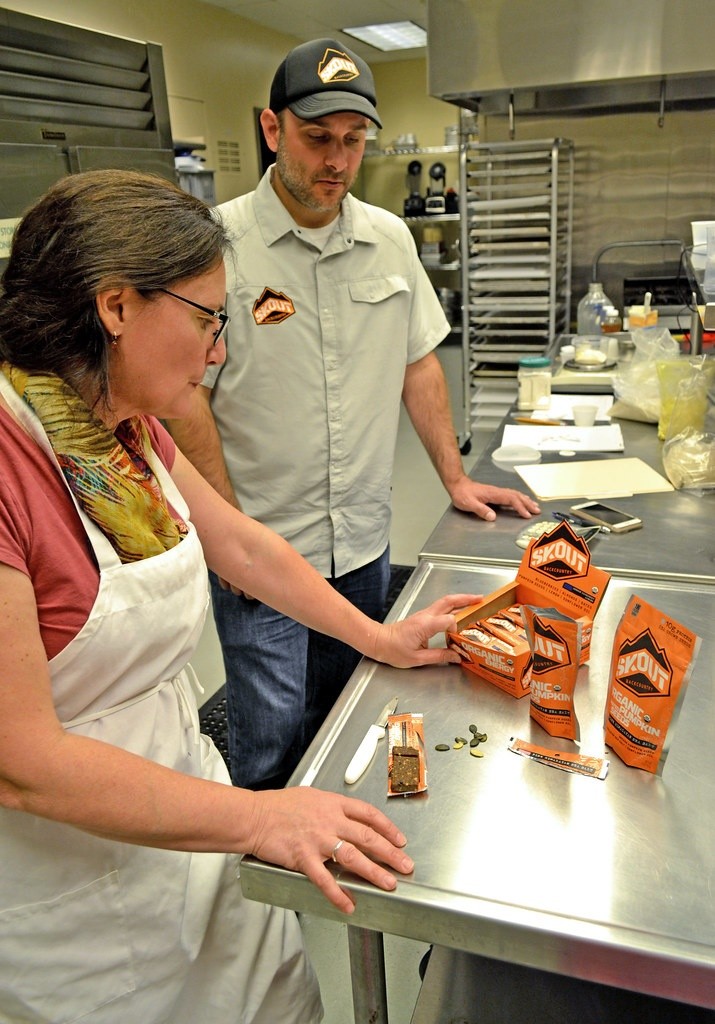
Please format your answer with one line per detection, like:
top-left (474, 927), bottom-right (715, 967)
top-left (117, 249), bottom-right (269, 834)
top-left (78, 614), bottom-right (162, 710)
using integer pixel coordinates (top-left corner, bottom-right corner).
top-left (548, 353), bottom-right (620, 394)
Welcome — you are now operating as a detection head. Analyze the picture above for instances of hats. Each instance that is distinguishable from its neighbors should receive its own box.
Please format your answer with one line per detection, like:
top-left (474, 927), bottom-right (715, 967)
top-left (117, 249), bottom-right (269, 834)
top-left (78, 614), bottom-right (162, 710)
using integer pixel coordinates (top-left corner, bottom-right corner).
top-left (269, 37), bottom-right (382, 130)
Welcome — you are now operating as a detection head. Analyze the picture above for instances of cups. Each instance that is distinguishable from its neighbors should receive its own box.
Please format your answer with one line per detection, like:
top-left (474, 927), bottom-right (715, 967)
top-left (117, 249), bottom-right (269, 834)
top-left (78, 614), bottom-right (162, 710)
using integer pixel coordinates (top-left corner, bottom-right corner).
top-left (690, 221), bottom-right (715, 246)
top-left (572, 404), bottom-right (598, 426)
top-left (703, 227), bottom-right (715, 294)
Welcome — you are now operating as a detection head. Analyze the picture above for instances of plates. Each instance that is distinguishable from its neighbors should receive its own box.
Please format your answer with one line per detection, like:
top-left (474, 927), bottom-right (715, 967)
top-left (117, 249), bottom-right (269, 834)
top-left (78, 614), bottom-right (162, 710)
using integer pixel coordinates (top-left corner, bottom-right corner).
top-left (443, 123), bottom-right (458, 146)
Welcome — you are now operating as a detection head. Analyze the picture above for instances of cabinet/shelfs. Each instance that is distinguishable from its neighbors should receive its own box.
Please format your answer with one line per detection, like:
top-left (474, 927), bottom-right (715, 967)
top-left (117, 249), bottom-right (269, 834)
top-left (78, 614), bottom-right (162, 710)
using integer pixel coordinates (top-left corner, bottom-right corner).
top-left (358, 146), bottom-right (462, 335)
top-left (459, 138), bottom-right (573, 454)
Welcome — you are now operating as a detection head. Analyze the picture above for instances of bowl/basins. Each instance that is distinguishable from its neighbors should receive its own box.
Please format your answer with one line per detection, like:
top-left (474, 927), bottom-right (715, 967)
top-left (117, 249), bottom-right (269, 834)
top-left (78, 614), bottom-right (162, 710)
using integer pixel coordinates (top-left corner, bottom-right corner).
top-left (437, 288), bottom-right (462, 326)
top-left (393, 132), bottom-right (417, 151)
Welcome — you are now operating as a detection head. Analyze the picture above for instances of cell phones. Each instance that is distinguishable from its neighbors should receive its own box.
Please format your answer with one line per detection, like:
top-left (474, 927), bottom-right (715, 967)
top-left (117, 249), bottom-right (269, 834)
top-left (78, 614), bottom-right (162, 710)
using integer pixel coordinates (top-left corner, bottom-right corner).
top-left (569, 501), bottom-right (642, 533)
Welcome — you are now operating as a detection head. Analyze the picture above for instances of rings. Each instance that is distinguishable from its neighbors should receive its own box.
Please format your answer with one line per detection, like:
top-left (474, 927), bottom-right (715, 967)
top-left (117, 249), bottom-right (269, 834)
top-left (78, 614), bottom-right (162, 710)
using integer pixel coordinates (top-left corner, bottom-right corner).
top-left (331, 839), bottom-right (345, 863)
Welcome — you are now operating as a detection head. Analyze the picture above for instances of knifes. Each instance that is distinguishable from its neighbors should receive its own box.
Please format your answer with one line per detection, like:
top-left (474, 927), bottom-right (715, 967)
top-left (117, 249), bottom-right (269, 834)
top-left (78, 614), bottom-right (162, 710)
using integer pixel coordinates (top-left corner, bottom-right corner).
top-left (343, 696), bottom-right (399, 784)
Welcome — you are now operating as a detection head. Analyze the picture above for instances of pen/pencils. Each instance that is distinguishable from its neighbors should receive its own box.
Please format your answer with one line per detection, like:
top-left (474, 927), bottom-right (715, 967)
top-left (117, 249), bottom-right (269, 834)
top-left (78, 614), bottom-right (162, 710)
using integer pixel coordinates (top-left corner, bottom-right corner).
top-left (551, 510), bottom-right (611, 534)
top-left (512, 417), bottom-right (567, 426)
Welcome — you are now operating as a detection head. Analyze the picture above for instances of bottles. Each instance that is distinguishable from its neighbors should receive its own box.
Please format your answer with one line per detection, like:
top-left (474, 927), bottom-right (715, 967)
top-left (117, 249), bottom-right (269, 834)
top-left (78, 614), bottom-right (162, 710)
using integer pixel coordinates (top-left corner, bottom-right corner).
top-left (517, 357), bottom-right (553, 411)
top-left (559, 345), bottom-right (576, 364)
top-left (598, 306), bottom-right (623, 333)
top-left (578, 283), bottom-right (616, 337)
top-left (445, 187), bottom-right (458, 214)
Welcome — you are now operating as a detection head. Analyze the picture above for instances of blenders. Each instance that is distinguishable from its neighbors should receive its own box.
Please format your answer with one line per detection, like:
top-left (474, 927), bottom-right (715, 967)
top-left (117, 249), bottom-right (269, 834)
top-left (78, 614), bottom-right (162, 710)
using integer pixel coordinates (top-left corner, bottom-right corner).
top-left (402, 160), bottom-right (426, 217)
top-left (424, 162), bottom-right (446, 216)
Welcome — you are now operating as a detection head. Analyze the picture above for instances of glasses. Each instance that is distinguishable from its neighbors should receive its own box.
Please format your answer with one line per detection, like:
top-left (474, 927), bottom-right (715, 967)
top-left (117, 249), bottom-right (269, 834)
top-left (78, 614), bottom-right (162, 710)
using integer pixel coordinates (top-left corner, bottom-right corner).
top-left (150, 283), bottom-right (232, 345)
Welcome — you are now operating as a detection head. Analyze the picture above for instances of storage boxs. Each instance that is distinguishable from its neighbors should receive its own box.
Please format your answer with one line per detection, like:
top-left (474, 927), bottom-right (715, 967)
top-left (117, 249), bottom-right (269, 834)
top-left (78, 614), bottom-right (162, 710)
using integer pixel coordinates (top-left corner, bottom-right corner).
top-left (445, 522), bottom-right (609, 698)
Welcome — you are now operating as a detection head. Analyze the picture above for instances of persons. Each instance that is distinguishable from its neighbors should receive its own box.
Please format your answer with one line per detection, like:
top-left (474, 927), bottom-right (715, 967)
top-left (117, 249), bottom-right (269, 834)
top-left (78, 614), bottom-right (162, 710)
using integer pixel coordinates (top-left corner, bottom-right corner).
top-left (158, 37), bottom-right (540, 792)
top-left (0, 168), bottom-right (482, 1024)
